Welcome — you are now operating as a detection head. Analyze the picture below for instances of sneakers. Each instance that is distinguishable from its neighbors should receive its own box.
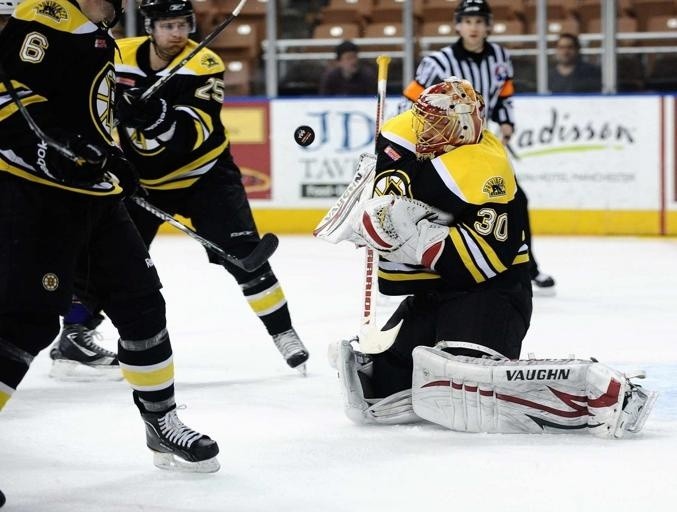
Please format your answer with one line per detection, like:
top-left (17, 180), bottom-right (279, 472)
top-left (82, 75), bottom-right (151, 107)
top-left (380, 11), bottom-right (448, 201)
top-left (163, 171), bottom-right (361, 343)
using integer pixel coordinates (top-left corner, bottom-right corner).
top-left (532, 272), bottom-right (556, 287)
top-left (271, 328), bottom-right (308, 367)
top-left (50, 326), bottom-right (123, 365)
top-left (132, 395), bottom-right (219, 463)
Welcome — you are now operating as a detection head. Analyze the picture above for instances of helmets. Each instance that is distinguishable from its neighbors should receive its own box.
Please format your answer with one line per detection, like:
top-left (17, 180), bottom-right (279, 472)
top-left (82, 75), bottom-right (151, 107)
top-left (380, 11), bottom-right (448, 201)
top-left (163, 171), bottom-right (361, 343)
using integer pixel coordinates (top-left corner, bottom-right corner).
top-left (409, 77), bottom-right (484, 159)
top-left (138, 0), bottom-right (197, 36)
top-left (454, 0), bottom-right (491, 24)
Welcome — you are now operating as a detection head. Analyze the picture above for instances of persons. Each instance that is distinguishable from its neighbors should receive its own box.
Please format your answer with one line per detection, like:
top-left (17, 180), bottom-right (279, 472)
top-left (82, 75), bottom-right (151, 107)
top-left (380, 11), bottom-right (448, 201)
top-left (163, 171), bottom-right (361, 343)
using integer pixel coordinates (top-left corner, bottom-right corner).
top-left (310, 76), bottom-right (652, 438)
top-left (48, 1), bottom-right (310, 383)
top-left (403, 1), bottom-right (555, 296)
top-left (0, 1), bottom-right (219, 511)
top-left (548, 32), bottom-right (602, 94)
top-left (320, 40), bottom-right (378, 98)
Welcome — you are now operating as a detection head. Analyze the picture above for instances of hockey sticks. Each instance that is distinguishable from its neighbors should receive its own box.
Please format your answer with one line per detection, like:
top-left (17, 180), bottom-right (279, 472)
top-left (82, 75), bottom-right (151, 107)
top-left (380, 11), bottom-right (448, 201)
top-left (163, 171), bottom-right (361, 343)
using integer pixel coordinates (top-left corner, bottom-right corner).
top-left (140, 0), bottom-right (245, 101)
top-left (1, 76), bottom-right (278, 274)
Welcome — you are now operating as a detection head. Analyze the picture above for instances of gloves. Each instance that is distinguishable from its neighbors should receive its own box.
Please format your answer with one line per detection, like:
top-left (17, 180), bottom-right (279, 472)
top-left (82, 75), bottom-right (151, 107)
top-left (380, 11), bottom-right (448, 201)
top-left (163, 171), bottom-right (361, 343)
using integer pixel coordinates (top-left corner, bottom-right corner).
top-left (118, 87), bottom-right (174, 137)
top-left (23, 126), bottom-right (107, 186)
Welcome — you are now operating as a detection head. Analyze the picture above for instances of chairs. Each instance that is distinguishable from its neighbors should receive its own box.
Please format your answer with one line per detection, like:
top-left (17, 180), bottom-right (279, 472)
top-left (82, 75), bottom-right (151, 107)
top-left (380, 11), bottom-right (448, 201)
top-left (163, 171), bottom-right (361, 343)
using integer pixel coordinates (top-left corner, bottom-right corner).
top-left (111, 0), bottom-right (676, 97)
